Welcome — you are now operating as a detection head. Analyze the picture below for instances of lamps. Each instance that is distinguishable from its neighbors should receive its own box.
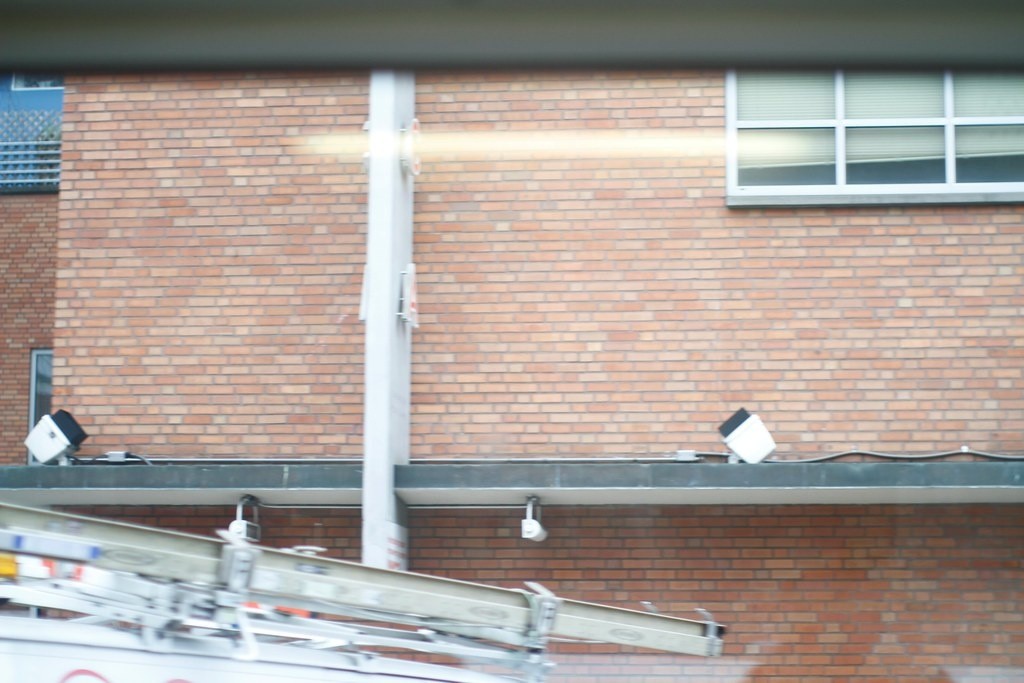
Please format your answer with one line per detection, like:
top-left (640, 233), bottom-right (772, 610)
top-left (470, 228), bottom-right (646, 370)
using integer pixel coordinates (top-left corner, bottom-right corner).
top-left (20, 408), bottom-right (90, 466)
top-left (225, 494), bottom-right (264, 546)
top-left (717, 408), bottom-right (778, 465)
top-left (522, 494), bottom-right (549, 545)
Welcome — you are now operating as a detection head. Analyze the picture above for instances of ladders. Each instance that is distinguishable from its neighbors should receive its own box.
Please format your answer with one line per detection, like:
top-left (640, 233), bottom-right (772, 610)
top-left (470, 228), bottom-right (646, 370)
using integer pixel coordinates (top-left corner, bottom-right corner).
top-left (0, 501), bottom-right (726, 661)
